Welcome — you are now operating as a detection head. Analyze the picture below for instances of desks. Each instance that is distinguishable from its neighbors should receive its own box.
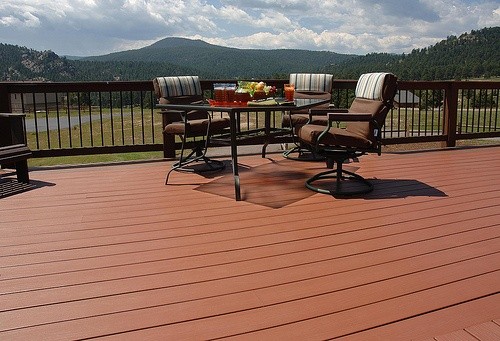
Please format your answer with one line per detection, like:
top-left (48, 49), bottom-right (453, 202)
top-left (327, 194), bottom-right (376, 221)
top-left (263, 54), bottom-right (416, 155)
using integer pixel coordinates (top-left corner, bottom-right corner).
top-left (154, 97), bottom-right (332, 202)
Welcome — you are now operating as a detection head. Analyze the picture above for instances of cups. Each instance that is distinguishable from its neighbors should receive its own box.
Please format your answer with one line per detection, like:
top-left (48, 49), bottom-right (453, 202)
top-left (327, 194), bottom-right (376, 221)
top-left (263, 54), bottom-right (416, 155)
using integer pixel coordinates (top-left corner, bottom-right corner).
top-left (226, 83), bottom-right (236, 102)
top-left (284, 84), bottom-right (296, 101)
top-left (213, 84), bottom-right (226, 102)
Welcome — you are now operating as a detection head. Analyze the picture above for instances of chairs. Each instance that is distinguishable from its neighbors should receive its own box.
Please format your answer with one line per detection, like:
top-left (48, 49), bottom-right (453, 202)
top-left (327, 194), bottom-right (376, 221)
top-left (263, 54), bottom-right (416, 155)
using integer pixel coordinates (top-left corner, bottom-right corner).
top-left (152, 74), bottom-right (232, 175)
top-left (282, 72), bottom-right (400, 198)
